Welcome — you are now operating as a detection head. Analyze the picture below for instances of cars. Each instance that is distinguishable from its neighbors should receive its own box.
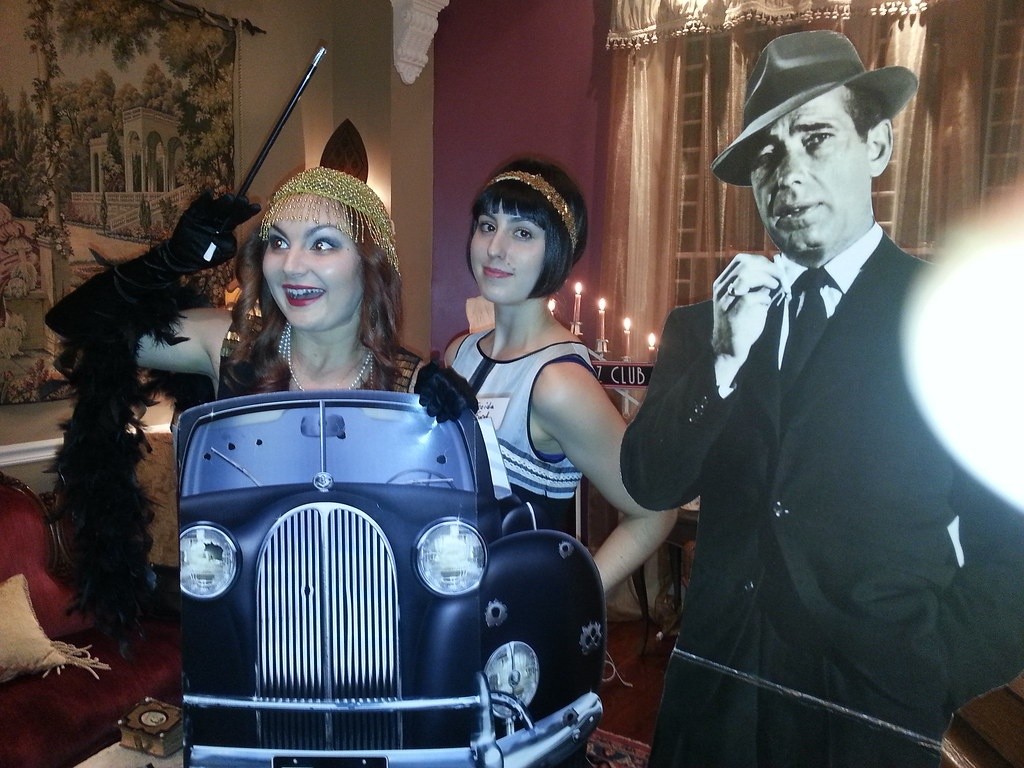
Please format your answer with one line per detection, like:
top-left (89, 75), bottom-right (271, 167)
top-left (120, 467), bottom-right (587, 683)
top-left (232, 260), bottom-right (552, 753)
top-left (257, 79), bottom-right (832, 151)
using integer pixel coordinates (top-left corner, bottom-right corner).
top-left (176, 387), bottom-right (607, 768)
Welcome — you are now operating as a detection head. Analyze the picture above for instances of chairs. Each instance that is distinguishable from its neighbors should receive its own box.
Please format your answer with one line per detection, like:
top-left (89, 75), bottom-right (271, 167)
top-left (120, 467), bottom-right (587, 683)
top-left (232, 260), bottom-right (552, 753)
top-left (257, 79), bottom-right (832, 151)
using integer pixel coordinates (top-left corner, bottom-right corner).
top-left (0, 473), bottom-right (181, 768)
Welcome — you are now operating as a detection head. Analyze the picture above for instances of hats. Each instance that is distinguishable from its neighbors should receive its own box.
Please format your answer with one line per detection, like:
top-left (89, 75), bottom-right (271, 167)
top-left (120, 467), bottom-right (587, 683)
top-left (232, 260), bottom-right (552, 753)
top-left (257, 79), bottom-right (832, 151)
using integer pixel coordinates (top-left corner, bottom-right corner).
top-left (259, 165), bottom-right (400, 276)
top-left (709, 30), bottom-right (916, 186)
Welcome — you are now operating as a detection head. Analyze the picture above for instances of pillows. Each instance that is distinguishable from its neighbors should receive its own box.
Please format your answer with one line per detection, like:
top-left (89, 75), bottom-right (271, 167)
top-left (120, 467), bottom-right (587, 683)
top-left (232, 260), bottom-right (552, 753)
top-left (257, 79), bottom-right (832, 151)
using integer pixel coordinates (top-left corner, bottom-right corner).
top-left (0, 574), bottom-right (112, 681)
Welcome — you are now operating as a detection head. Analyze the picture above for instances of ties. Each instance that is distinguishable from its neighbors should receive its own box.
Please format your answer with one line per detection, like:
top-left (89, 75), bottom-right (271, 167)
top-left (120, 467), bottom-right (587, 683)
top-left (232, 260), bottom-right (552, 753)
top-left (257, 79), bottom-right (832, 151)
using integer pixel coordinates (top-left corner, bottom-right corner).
top-left (779, 268), bottom-right (834, 399)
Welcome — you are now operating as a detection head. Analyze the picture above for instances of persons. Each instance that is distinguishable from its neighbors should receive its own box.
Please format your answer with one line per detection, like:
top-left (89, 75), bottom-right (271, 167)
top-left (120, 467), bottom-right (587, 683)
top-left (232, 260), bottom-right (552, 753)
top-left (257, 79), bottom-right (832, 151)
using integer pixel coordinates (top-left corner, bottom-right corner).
top-left (45, 167), bottom-right (478, 423)
top-left (444, 156), bottom-right (678, 594)
top-left (619, 29), bottom-right (1024, 768)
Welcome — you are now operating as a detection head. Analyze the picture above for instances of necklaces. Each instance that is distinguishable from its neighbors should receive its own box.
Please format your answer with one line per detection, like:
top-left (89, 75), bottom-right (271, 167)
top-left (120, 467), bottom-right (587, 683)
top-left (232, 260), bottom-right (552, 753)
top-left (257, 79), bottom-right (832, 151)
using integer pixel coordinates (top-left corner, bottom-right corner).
top-left (278, 322), bottom-right (374, 392)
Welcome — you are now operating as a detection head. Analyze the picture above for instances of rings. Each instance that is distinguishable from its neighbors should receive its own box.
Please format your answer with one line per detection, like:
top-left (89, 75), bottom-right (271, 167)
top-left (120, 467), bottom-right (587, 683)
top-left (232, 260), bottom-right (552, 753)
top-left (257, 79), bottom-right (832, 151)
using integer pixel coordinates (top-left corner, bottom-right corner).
top-left (728, 283), bottom-right (750, 295)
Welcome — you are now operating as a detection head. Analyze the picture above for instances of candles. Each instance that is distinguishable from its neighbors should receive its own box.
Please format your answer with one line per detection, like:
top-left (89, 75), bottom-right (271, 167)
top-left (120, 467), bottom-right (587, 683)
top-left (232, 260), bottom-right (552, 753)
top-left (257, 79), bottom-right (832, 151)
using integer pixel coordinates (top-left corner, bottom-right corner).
top-left (569, 282), bottom-right (583, 336)
top-left (595, 297), bottom-right (609, 361)
top-left (621, 316), bottom-right (633, 363)
top-left (647, 332), bottom-right (656, 363)
top-left (548, 299), bottom-right (556, 316)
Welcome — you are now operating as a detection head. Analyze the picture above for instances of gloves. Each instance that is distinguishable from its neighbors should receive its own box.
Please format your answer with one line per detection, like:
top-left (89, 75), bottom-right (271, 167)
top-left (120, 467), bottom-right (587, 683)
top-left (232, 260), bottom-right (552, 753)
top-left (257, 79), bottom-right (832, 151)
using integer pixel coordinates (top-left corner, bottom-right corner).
top-left (413, 351), bottom-right (480, 424)
top-left (148, 190), bottom-right (262, 275)
